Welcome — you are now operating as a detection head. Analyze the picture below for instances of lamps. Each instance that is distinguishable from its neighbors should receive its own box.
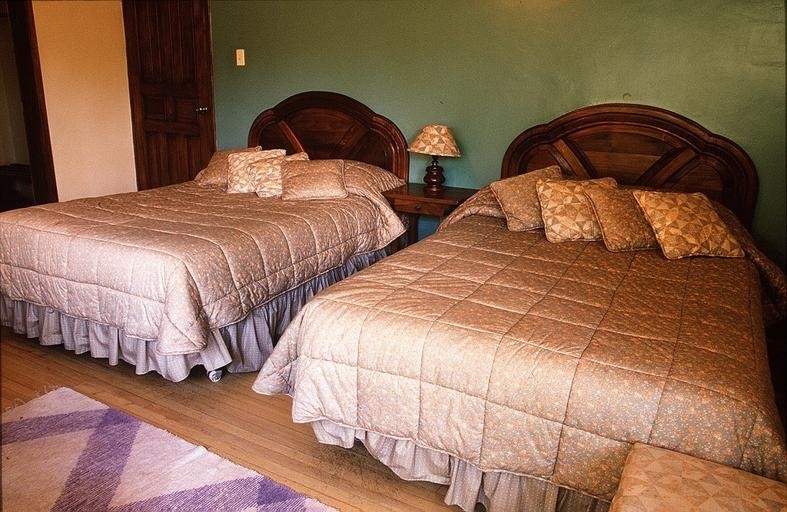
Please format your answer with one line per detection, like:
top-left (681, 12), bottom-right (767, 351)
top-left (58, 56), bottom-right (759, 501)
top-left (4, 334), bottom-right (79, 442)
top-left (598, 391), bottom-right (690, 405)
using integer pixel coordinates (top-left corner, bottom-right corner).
top-left (407, 124), bottom-right (462, 193)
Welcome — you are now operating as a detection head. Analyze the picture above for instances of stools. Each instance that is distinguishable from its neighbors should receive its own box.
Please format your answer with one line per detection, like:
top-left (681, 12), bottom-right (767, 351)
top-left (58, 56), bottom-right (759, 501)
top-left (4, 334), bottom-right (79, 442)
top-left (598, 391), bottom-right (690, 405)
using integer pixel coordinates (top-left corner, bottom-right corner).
top-left (602, 442), bottom-right (786, 511)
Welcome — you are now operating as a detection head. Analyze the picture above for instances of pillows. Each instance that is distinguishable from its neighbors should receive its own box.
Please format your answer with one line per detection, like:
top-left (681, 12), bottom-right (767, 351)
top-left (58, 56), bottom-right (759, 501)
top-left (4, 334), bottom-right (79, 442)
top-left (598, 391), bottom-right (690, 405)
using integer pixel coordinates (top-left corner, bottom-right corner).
top-left (224, 148), bottom-right (286, 195)
top-left (279, 157), bottom-right (350, 204)
top-left (488, 162), bottom-right (562, 235)
top-left (344, 158), bottom-right (408, 193)
top-left (583, 185), bottom-right (657, 252)
top-left (631, 184), bottom-right (745, 264)
top-left (248, 151), bottom-right (309, 198)
top-left (532, 176), bottom-right (619, 245)
top-left (199, 144), bottom-right (262, 186)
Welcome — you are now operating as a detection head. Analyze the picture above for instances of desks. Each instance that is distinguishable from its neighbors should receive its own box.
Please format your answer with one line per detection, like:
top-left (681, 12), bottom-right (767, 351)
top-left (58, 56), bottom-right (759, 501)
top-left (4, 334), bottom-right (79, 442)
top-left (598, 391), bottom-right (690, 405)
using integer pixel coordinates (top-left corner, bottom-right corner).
top-left (380, 181), bottom-right (480, 257)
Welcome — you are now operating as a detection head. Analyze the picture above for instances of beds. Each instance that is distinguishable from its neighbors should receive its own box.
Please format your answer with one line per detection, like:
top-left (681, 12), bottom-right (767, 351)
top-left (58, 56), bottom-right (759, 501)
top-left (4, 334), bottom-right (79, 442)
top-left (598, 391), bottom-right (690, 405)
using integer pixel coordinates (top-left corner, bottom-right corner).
top-left (249, 101), bottom-right (785, 511)
top-left (0, 88), bottom-right (413, 384)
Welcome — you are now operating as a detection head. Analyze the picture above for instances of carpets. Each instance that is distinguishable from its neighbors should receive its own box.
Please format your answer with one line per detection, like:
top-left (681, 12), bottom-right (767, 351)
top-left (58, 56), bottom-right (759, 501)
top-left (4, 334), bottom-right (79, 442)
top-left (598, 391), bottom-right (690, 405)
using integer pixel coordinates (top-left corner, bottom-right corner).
top-left (0, 387), bottom-right (335, 512)
